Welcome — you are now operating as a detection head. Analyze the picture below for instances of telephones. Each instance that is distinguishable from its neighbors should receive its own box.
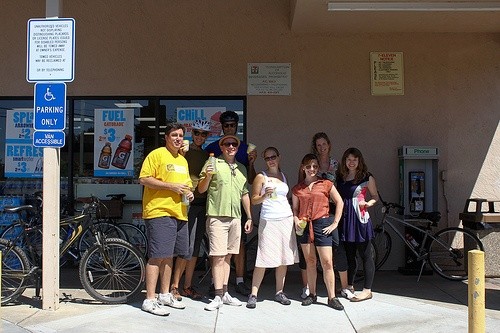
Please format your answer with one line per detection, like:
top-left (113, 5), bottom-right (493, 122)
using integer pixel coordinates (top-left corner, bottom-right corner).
top-left (408, 170), bottom-right (425, 198)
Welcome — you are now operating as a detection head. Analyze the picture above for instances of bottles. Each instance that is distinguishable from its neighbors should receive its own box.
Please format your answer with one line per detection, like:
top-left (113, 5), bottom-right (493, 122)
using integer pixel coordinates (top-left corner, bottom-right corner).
top-left (295, 217), bottom-right (307, 236)
top-left (268, 180), bottom-right (277, 200)
top-left (34, 157), bottom-right (43, 173)
top-left (98, 142), bottom-right (112, 168)
top-left (182, 184), bottom-right (190, 206)
top-left (405, 233), bottom-right (420, 249)
top-left (209, 152), bottom-right (217, 174)
top-left (111, 135), bottom-right (133, 169)
top-left (322, 172), bottom-right (336, 182)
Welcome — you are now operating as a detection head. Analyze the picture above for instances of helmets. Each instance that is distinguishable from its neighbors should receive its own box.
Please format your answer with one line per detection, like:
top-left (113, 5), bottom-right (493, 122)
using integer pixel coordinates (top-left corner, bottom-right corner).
top-left (190, 119), bottom-right (213, 132)
top-left (219, 110), bottom-right (239, 124)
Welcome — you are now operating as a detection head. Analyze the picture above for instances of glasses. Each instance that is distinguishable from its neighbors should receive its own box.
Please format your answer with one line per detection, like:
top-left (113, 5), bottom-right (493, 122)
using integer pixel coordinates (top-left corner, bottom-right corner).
top-left (222, 123), bottom-right (237, 128)
top-left (192, 130), bottom-right (208, 136)
top-left (222, 142), bottom-right (238, 147)
top-left (306, 165), bottom-right (319, 169)
top-left (264, 155), bottom-right (278, 161)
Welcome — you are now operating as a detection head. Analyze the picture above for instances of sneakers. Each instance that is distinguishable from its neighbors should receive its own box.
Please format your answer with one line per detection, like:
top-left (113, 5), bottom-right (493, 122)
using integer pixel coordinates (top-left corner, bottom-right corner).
top-left (158, 292), bottom-right (186, 309)
top-left (141, 298), bottom-right (170, 316)
top-left (221, 291), bottom-right (242, 306)
top-left (246, 294), bottom-right (257, 308)
top-left (204, 295), bottom-right (224, 311)
top-left (274, 293), bottom-right (291, 305)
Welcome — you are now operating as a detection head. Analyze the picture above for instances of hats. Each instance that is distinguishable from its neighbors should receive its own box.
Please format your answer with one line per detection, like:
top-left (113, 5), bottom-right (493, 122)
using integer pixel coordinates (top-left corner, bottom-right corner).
top-left (219, 134), bottom-right (241, 152)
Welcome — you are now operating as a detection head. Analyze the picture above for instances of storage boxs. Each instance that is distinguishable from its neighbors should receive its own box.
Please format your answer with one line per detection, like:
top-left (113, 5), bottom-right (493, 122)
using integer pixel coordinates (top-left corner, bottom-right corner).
top-left (96, 200), bottom-right (123, 218)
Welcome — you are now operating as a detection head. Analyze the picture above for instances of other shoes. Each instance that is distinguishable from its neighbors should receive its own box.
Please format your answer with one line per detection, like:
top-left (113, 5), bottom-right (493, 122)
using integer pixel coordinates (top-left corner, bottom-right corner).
top-left (169, 283), bottom-right (183, 301)
top-left (301, 286), bottom-right (310, 299)
top-left (327, 297), bottom-right (344, 310)
top-left (302, 293), bottom-right (317, 306)
top-left (235, 283), bottom-right (251, 296)
top-left (341, 288), bottom-right (353, 297)
top-left (350, 292), bottom-right (372, 302)
top-left (181, 286), bottom-right (202, 299)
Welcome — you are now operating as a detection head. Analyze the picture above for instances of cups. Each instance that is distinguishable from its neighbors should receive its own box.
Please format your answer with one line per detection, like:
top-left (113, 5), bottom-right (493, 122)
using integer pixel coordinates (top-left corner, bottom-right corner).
top-left (247, 143), bottom-right (256, 154)
top-left (182, 139), bottom-right (189, 152)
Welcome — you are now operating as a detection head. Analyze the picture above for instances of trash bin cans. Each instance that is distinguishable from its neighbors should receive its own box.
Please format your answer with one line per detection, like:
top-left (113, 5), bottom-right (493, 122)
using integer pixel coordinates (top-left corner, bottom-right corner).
top-left (459, 197), bottom-right (500, 278)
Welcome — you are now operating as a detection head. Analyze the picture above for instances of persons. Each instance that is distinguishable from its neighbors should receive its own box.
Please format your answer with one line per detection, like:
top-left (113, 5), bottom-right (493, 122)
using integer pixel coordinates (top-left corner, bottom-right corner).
top-left (203, 110), bottom-right (256, 298)
top-left (197, 134), bottom-right (254, 310)
top-left (138, 122), bottom-right (203, 321)
top-left (167, 119), bottom-right (214, 301)
top-left (245, 147), bottom-right (301, 308)
top-left (290, 153), bottom-right (345, 311)
top-left (297, 132), bottom-right (357, 299)
top-left (334, 148), bottom-right (380, 301)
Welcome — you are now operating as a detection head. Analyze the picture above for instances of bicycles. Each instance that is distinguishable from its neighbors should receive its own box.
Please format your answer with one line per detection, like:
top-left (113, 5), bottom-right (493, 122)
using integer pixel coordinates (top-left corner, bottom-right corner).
top-left (0, 189), bottom-right (149, 307)
top-left (245, 223), bottom-right (377, 284)
top-left (355, 191), bottom-right (484, 283)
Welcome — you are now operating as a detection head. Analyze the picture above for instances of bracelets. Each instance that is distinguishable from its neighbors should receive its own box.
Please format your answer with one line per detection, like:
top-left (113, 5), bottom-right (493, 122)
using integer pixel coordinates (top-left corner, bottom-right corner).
top-left (247, 218), bottom-right (253, 220)
